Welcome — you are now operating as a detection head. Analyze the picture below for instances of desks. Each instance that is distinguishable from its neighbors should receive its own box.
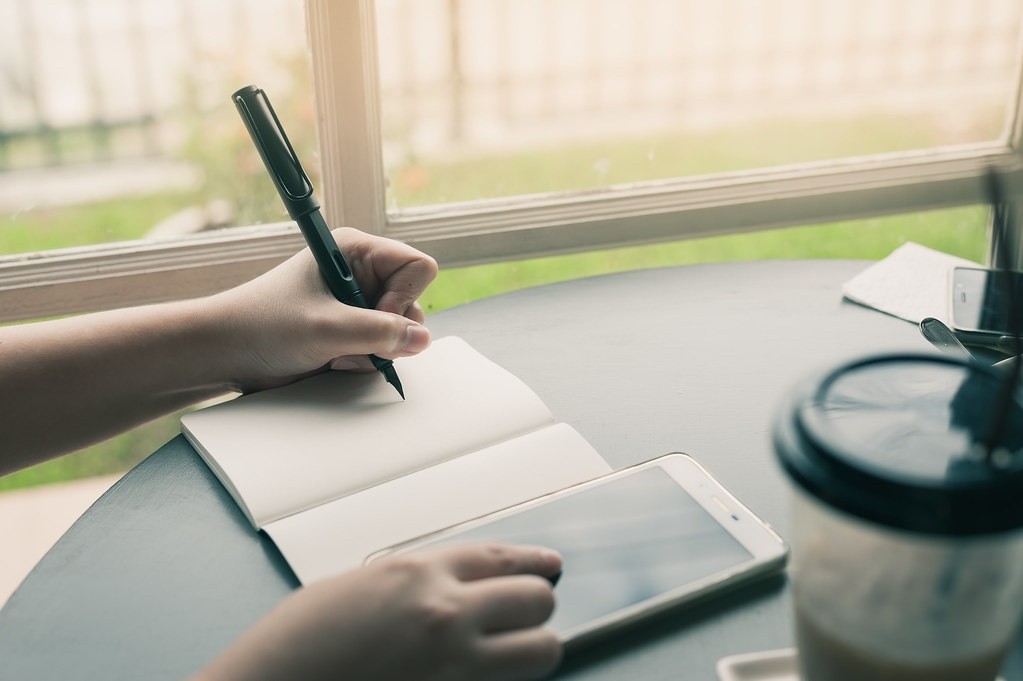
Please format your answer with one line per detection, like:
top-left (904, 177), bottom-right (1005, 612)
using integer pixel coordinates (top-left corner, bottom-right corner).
top-left (0, 260), bottom-right (1023, 681)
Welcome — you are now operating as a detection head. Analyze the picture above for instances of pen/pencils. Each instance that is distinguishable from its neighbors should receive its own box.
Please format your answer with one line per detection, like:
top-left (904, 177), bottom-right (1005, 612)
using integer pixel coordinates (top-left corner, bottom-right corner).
top-left (230, 83), bottom-right (406, 401)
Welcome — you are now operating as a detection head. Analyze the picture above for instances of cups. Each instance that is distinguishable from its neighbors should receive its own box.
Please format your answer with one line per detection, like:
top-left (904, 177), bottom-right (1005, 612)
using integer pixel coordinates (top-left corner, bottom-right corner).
top-left (768, 348), bottom-right (1023, 681)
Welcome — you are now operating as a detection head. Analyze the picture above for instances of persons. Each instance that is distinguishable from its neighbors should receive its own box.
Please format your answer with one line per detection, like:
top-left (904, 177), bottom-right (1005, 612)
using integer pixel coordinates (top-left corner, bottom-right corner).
top-left (0, 227), bottom-right (563, 681)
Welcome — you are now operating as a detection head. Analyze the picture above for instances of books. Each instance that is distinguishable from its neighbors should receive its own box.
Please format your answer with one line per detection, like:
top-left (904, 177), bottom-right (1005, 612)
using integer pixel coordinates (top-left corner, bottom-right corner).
top-left (181, 337), bottom-right (618, 589)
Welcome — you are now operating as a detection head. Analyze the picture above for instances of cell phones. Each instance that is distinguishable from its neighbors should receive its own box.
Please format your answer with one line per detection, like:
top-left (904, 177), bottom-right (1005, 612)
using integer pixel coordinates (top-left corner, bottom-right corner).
top-left (947, 266), bottom-right (1023, 349)
top-left (360, 451), bottom-right (793, 654)
